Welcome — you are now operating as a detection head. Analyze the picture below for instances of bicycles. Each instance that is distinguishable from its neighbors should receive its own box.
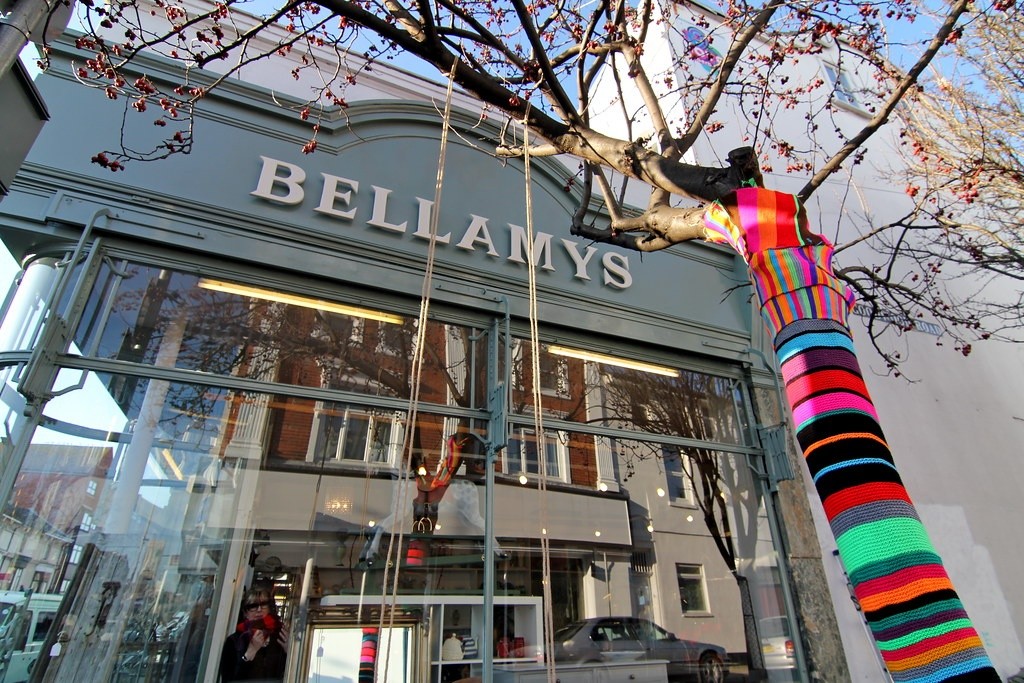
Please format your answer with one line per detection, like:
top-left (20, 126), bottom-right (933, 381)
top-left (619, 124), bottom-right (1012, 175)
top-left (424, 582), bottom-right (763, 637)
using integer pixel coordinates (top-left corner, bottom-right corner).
top-left (109, 601), bottom-right (184, 683)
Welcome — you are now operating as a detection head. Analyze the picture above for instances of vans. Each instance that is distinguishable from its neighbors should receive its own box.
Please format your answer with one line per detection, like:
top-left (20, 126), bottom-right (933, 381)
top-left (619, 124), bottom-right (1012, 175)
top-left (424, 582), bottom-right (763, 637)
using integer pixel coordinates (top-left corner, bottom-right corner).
top-left (751, 616), bottom-right (799, 672)
top-left (1, 590), bottom-right (65, 682)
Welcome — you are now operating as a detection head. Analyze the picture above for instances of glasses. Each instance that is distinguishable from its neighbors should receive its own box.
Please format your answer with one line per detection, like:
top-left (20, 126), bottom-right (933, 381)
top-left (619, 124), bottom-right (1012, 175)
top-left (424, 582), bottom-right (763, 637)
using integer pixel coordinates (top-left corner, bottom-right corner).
top-left (248, 600), bottom-right (270, 611)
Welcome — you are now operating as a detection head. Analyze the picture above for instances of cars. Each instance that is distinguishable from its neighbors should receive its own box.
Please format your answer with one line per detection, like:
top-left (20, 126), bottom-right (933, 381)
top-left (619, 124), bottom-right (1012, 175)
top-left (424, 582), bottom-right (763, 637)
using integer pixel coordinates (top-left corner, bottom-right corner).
top-left (481, 617), bottom-right (730, 683)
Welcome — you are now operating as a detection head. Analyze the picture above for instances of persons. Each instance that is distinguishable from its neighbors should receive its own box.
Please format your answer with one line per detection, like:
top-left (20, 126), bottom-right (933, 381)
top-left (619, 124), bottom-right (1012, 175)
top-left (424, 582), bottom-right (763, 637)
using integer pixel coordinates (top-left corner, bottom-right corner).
top-left (219, 586), bottom-right (287, 683)
